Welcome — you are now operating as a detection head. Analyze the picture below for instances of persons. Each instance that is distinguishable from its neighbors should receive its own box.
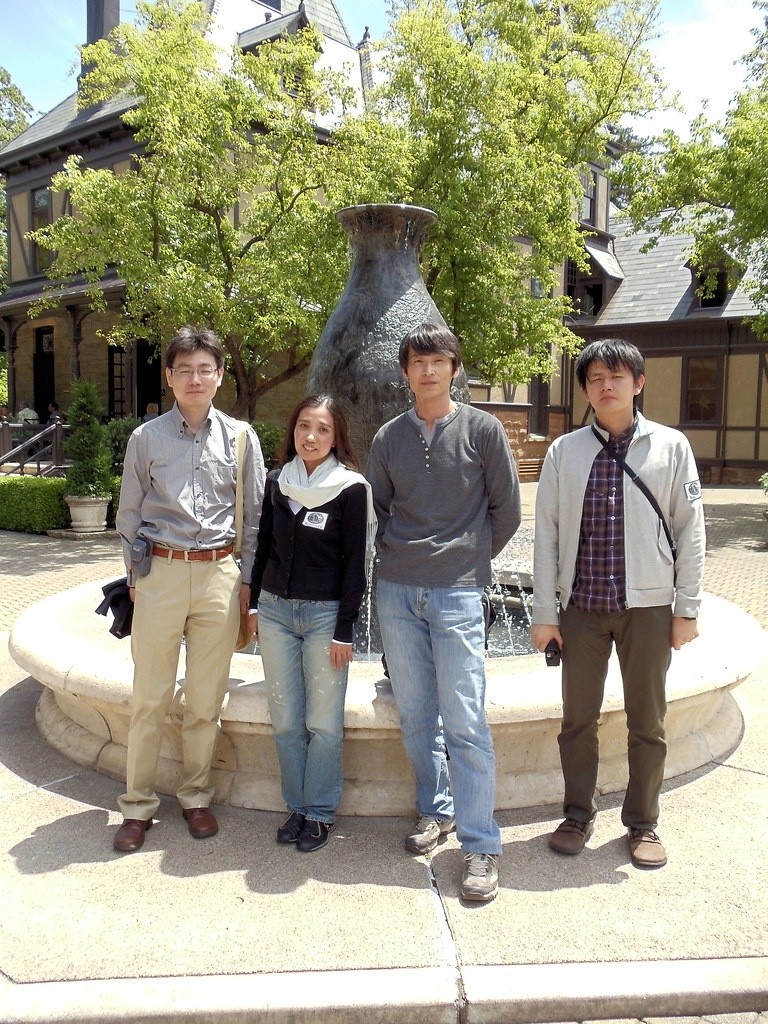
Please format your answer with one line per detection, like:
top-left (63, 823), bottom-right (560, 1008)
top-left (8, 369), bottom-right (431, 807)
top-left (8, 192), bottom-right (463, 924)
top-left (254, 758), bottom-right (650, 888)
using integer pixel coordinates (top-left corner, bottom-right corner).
top-left (114, 325), bottom-right (266, 855)
top-left (0, 405), bottom-right (8, 416)
top-left (248, 396), bottom-right (378, 852)
top-left (143, 403), bottom-right (158, 422)
top-left (16, 401), bottom-right (39, 437)
top-left (46, 402), bottom-right (64, 424)
top-left (532, 340), bottom-right (706, 868)
top-left (368, 322), bottom-right (521, 900)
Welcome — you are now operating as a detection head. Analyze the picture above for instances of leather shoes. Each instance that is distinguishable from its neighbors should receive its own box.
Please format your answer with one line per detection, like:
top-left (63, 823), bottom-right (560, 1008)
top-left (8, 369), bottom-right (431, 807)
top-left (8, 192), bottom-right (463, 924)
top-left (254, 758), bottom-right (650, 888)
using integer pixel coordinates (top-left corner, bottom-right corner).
top-left (113, 817), bottom-right (153, 851)
top-left (628, 827), bottom-right (667, 868)
top-left (548, 817), bottom-right (594, 855)
top-left (182, 808), bottom-right (218, 839)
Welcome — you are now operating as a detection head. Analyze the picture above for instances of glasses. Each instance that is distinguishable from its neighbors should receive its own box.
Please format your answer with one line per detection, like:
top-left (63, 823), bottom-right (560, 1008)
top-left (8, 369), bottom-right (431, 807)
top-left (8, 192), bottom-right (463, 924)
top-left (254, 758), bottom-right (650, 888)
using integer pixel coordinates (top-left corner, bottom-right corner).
top-left (170, 368), bottom-right (218, 383)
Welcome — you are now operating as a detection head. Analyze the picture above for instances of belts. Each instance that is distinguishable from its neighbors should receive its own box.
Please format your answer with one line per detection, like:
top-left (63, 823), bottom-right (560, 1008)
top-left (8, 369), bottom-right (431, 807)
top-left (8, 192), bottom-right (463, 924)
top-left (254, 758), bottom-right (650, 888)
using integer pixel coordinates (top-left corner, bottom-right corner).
top-left (153, 543), bottom-right (233, 562)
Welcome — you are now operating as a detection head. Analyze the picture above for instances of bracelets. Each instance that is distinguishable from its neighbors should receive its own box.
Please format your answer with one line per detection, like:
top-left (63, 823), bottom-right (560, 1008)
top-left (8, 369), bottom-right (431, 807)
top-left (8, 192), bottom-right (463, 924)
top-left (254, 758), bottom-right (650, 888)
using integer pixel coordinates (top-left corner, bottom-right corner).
top-left (681, 617), bottom-right (697, 621)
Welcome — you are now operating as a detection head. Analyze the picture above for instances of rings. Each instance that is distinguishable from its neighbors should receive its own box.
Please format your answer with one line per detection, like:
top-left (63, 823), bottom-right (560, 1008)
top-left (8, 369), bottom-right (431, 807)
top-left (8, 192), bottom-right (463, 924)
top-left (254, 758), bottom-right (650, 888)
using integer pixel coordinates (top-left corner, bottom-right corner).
top-left (253, 631), bottom-right (257, 635)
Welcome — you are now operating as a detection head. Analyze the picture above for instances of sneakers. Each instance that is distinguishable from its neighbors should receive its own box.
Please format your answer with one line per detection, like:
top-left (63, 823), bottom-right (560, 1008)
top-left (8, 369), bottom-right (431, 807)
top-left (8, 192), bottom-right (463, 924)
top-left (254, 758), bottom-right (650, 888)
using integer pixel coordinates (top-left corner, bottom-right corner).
top-left (461, 852), bottom-right (499, 900)
top-left (296, 820), bottom-right (337, 852)
top-left (405, 814), bottom-right (456, 855)
top-left (277, 811), bottom-right (306, 842)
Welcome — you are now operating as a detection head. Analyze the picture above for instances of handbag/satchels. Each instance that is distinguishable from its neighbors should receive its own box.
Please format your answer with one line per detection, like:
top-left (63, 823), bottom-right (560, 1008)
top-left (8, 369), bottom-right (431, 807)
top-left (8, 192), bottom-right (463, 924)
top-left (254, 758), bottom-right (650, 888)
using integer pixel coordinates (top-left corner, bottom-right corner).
top-left (234, 603), bottom-right (252, 651)
